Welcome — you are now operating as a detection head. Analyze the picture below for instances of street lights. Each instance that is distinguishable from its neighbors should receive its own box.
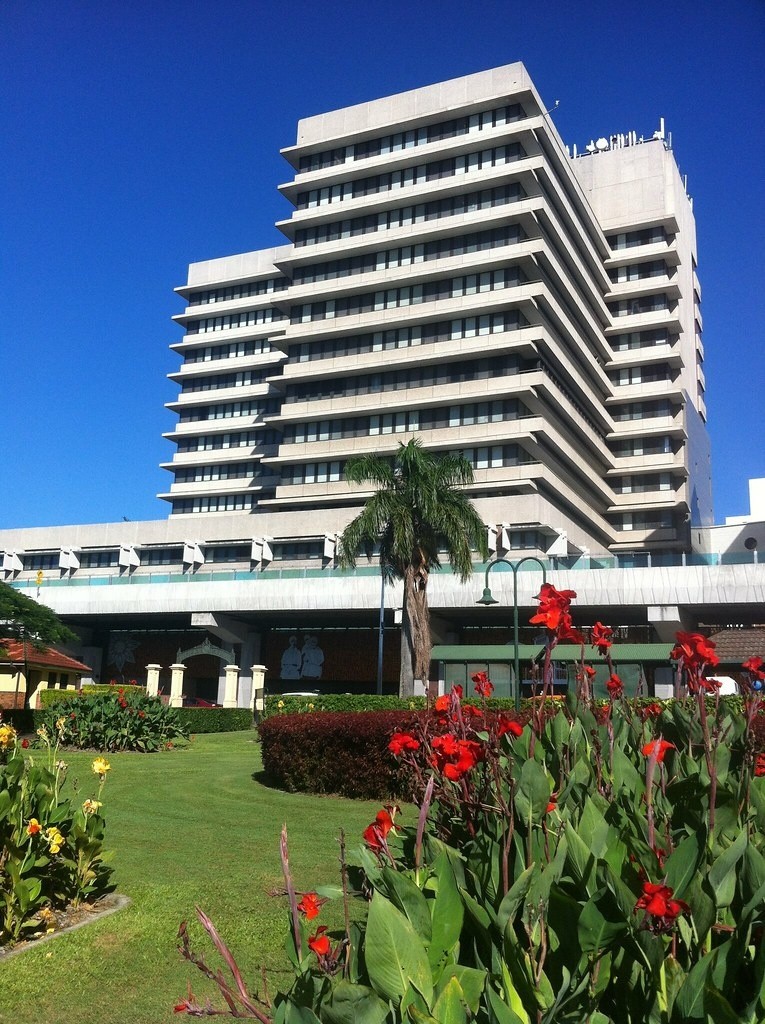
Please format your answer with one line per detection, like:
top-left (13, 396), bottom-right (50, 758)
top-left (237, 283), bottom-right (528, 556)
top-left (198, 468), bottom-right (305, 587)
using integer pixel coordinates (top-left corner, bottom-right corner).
top-left (476, 557), bottom-right (546, 712)
